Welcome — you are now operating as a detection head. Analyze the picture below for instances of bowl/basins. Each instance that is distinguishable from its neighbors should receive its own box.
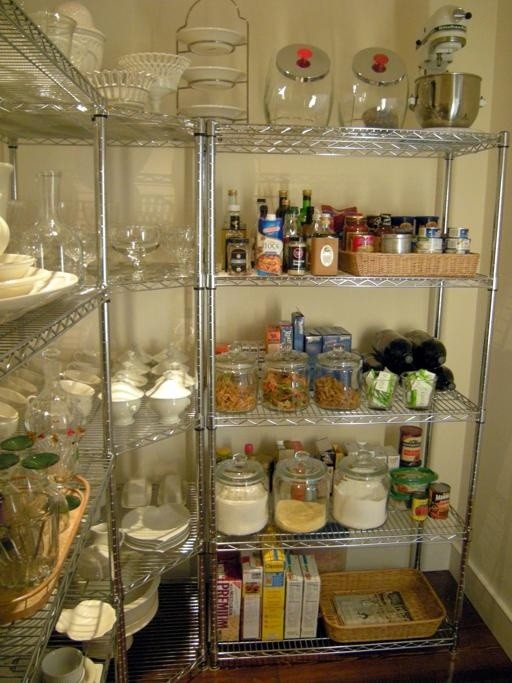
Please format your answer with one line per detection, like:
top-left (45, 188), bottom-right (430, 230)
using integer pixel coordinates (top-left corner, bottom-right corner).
top-left (116, 574), bottom-right (161, 653)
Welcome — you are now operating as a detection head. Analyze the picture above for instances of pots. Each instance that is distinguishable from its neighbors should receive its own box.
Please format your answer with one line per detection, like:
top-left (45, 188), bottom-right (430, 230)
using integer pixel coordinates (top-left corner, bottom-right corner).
top-left (411, 73), bottom-right (486, 127)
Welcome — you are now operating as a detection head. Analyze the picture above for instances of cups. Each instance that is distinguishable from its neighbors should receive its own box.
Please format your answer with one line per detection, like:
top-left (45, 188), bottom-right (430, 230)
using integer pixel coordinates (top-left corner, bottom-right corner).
top-left (25, 10), bottom-right (76, 60)
top-left (42, 647), bottom-right (84, 683)
top-left (0, 344), bottom-right (197, 445)
top-left (364, 369), bottom-right (438, 410)
top-left (91, 521), bottom-right (125, 554)
top-left (3, 491), bottom-right (59, 586)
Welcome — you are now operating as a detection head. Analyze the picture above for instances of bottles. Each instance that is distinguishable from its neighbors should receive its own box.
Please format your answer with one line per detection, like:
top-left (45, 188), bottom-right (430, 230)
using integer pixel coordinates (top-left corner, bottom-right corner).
top-left (221, 189), bottom-right (470, 277)
top-left (338, 47), bottom-right (409, 128)
top-left (215, 425), bottom-right (451, 537)
top-left (216, 341), bottom-right (362, 414)
top-left (26, 348), bottom-right (85, 476)
top-left (361, 329), bottom-right (456, 392)
top-left (264, 43), bottom-right (334, 125)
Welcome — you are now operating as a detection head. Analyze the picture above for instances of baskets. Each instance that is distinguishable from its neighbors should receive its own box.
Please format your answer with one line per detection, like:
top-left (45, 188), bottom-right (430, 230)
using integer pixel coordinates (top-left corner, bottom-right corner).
top-left (320, 568), bottom-right (446, 642)
top-left (339, 252), bottom-right (480, 278)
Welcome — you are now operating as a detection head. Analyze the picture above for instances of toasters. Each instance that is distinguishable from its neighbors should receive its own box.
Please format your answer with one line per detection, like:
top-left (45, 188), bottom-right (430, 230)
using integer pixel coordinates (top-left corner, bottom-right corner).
top-left (1, 161), bottom-right (96, 290)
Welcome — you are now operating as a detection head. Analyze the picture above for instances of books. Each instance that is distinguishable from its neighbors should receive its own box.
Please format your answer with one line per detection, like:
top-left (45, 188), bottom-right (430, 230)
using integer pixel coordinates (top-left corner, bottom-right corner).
top-left (331, 590), bottom-right (413, 625)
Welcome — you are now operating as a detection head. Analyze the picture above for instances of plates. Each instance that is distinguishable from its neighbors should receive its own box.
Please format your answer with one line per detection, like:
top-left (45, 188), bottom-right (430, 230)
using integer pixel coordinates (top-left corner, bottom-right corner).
top-left (121, 503), bottom-right (192, 552)
top-left (176, 27), bottom-right (241, 53)
top-left (180, 65), bottom-right (243, 90)
top-left (0, 253), bottom-right (78, 318)
top-left (178, 104), bottom-right (246, 124)
top-left (122, 477), bottom-right (152, 507)
top-left (57, 599), bottom-right (116, 641)
top-left (158, 469), bottom-right (189, 504)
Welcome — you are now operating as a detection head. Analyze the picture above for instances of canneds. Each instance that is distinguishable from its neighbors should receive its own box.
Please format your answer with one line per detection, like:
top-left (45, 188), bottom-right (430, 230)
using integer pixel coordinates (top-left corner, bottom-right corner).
top-left (427, 483), bottom-right (451, 519)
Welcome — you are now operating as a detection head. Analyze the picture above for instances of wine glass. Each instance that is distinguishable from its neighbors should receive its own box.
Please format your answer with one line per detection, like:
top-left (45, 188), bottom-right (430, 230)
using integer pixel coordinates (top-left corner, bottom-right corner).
top-left (113, 223), bottom-right (162, 272)
top-left (165, 224), bottom-right (198, 278)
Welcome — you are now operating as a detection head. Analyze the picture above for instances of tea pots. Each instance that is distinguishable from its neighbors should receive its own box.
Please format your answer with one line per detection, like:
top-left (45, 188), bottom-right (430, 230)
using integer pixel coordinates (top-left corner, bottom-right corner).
top-left (78, 544), bottom-right (111, 582)
